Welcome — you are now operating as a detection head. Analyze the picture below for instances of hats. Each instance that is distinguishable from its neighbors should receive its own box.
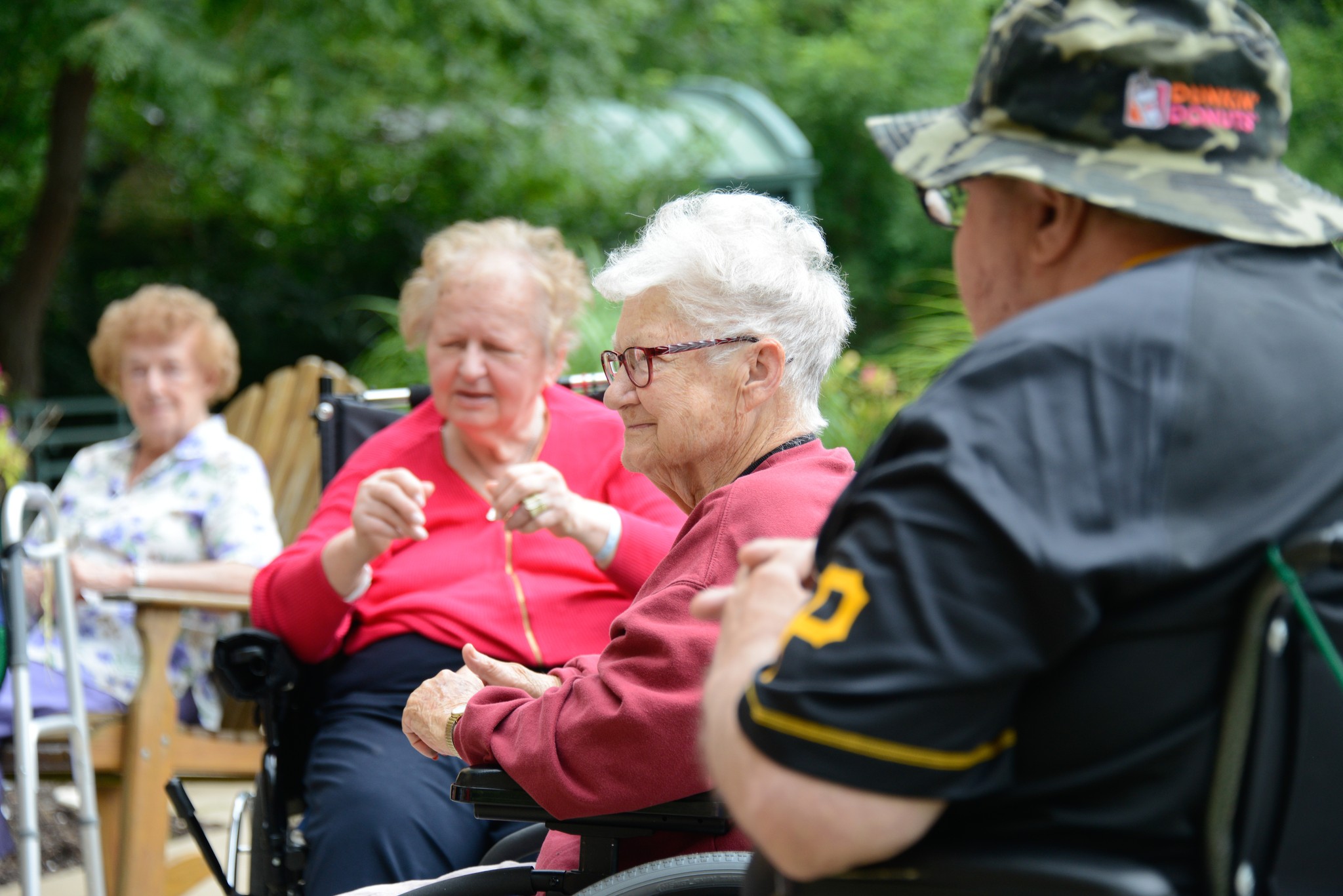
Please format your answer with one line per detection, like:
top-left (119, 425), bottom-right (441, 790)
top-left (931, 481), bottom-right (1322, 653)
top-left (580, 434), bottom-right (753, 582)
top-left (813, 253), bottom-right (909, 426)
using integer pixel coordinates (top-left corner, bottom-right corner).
top-left (864, 1), bottom-right (1343, 246)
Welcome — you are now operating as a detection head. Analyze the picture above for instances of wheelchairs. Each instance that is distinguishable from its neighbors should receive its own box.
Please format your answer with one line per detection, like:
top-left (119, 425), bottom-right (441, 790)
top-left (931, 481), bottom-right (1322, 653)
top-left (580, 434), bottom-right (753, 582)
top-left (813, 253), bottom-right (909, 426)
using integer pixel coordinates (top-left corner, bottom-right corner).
top-left (165, 373), bottom-right (1343, 895)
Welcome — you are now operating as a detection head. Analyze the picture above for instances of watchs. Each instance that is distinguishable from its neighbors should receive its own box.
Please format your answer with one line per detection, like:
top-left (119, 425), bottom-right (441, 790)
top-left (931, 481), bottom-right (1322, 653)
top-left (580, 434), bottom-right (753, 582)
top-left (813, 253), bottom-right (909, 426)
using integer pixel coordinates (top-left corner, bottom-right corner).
top-left (445, 702), bottom-right (469, 760)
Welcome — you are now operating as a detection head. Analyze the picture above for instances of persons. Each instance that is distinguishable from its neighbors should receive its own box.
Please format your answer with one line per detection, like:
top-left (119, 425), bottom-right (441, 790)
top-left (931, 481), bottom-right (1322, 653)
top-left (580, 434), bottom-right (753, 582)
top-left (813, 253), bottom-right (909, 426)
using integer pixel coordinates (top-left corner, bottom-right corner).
top-left (0, 283), bottom-right (285, 860)
top-left (689, 0), bottom-right (1342, 896)
top-left (332, 180), bottom-right (857, 896)
top-left (250, 215), bottom-right (688, 896)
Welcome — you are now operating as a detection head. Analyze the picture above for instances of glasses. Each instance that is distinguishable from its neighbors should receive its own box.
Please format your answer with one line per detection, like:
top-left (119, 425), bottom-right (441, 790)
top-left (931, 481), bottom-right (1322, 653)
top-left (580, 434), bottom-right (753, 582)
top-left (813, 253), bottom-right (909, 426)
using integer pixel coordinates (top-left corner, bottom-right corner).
top-left (915, 177), bottom-right (982, 228)
top-left (600, 336), bottom-right (758, 387)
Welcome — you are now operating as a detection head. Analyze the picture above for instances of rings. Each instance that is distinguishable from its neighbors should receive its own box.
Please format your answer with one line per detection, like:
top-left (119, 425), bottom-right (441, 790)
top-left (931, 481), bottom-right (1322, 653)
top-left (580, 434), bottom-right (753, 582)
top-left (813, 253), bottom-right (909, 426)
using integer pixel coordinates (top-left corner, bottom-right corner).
top-left (523, 492), bottom-right (546, 518)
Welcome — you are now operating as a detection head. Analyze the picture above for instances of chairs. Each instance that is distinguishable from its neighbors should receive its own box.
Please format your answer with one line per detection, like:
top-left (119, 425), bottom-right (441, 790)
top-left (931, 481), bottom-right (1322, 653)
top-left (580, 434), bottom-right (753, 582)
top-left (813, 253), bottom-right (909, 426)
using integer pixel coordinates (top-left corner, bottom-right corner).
top-left (0, 357), bottom-right (368, 896)
